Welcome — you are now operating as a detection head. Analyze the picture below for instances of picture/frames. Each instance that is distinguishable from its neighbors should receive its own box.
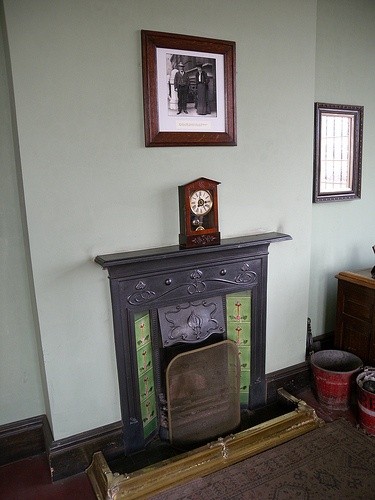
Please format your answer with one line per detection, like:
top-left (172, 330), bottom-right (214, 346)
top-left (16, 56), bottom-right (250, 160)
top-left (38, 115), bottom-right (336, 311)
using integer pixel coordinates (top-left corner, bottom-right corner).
top-left (141, 30), bottom-right (237, 147)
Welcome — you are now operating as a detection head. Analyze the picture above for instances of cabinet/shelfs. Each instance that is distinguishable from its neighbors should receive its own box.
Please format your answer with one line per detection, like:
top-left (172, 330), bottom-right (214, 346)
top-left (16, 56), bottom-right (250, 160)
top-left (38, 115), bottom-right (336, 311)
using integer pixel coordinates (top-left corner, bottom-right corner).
top-left (333, 279), bottom-right (375, 367)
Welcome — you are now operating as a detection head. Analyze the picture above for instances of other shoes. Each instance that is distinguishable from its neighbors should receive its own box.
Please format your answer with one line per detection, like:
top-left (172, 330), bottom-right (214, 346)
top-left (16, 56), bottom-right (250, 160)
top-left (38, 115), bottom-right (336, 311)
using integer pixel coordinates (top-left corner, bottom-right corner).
top-left (177, 110), bottom-right (181, 114)
top-left (184, 111), bottom-right (188, 114)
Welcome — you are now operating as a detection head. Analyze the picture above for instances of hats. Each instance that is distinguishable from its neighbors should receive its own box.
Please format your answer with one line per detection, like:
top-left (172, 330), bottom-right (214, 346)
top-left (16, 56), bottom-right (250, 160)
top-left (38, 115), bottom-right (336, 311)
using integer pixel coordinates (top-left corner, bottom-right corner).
top-left (177, 63), bottom-right (185, 67)
top-left (194, 63), bottom-right (205, 67)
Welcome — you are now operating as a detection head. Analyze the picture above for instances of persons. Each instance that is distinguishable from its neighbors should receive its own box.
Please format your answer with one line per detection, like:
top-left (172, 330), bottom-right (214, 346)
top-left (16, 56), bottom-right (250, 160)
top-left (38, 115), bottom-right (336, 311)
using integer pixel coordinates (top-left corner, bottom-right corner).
top-left (195, 64), bottom-right (210, 114)
top-left (174, 62), bottom-right (189, 115)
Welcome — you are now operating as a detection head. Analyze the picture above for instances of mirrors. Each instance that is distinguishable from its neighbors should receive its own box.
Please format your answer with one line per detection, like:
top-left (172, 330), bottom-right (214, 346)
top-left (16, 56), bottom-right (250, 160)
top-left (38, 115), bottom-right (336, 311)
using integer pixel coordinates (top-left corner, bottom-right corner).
top-left (313, 101), bottom-right (364, 204)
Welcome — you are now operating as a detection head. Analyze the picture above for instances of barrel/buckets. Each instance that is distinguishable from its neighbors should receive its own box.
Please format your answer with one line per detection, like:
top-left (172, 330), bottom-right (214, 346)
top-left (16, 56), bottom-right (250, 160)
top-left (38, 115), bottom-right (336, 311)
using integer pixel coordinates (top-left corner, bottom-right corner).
top-left (356, 366), bottom-right (375, 436)
top-left (310, 350), bottom-right (363, 410)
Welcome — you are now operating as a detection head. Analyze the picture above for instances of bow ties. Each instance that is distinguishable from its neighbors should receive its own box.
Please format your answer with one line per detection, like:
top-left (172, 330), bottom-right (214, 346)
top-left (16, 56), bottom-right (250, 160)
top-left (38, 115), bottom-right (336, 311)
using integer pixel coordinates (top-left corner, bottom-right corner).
top-left (181, 71), bottom-right (183, 72)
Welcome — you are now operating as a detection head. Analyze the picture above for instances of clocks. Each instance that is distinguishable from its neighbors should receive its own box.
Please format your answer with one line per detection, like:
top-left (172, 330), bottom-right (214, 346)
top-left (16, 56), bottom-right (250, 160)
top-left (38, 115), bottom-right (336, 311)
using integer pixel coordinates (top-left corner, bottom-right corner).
top-left (178, 177), bottom-right (221, 250)
